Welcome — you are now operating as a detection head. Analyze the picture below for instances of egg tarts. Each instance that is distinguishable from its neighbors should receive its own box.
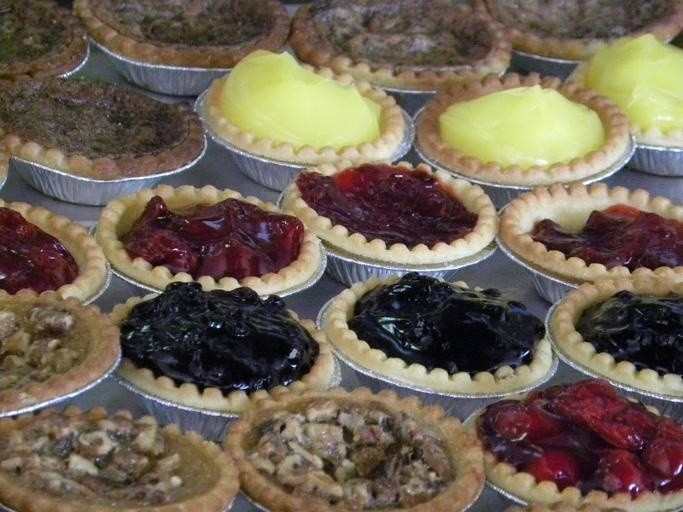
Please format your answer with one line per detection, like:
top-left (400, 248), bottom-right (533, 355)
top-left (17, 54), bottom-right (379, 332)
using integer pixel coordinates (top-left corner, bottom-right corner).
top-left (204, 48), bottom-right (404, 168)
top-left (548, 279), bottom-right (683, 404)
top-left (69, 1), bottom-right (290, 68)
top-left (497, 182), bottom-right (682, 290)
top-left (1, 198), bottom-right (109, 300)
top-left (282, 159), bottom-right (500, 266)
top-left (324, 272), bottom-right (556, 389)
top-left (2, 289), bottom-right (122, 419)
top-left (103, 280), bottom-right (335, 413)
top-left (1, 130), bottom-right (11, 193)
top-left (472, 380), bottom-right (682, 510)
top-left (568, 32), bottom-right (682, 155)
top-left (233, 383), bottom-right (488, 510)
top-left (415, 71), bottom-right (639, 189)
top-left (472, 1), bottom-right (681, 68)
top-left (0, 1), bottom-right (91, 84)
top-left (1, 403), bottom-right (241, 510)
top-left (5, 79), bottom-right (206, 180)
top-left (290, 1), bottom-right (513, 92)
top-left (97, 184), bottom-right (325, 297)
